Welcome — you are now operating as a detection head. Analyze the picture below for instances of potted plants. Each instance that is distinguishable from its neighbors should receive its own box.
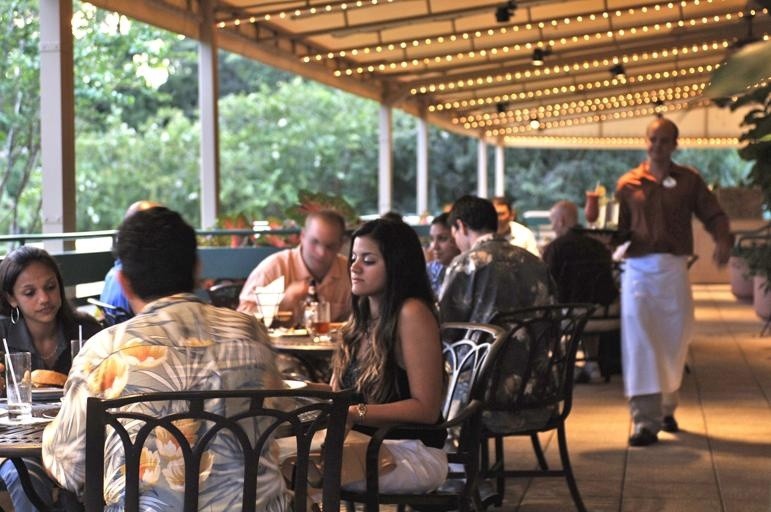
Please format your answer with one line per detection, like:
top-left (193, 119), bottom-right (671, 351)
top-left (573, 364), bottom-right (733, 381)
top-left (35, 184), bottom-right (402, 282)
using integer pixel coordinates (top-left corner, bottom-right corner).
top-left (747, 245), bottom-right (770, 324)
top-left (728, 245), bottom-right (755, 302)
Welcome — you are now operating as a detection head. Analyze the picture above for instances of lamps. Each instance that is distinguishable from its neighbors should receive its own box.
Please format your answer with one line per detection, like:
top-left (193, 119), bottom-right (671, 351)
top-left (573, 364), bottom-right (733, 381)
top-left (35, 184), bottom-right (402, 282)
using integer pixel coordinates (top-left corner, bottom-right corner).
top-left (531, 46), bottom-right (551, 65)
top-left (491, 2), bottom-right (519, 25)
top-left (495, 101), bottom-right (505, 119)
top-left (609, 62), bottom-right (628, 80)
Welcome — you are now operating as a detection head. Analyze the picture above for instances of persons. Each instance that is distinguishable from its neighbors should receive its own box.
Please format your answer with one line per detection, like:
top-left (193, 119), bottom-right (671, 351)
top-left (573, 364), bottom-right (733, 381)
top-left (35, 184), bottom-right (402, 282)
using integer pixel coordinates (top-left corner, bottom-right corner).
top-left (606, 117), bottom-right (733, 447)
top-left (0, 246), bottom-right (109, 511)
top-left (237, 206), bottom-right (448, 511)
top-left (42, 205), bottom-right (293, 512)
top-left (426, 195), bottom-right (614, 510)
top-left (94, 201), bottom-right (167, 321)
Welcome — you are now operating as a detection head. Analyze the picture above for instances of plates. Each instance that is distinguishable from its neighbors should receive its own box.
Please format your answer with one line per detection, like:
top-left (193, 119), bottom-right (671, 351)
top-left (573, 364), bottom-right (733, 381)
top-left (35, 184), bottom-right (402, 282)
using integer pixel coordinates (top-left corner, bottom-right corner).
top-left (282, 378), bottom-right (308, 391)
top-left (30, 388), bottom-right (67, 401)
top-left (278, 329), bottom-right (309, 338)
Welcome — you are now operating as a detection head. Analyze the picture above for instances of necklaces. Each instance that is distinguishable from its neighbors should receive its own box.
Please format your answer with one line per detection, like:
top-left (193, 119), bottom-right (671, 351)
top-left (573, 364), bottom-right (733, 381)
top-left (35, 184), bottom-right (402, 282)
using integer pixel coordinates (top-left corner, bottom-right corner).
top-left (39, 342), bottom-right (59, 361)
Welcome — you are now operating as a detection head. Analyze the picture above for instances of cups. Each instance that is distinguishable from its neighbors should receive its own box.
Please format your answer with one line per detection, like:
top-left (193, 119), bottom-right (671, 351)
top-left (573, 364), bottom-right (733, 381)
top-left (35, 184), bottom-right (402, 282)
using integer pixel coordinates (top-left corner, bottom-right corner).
top-left (69, 339), bottom-right (84, 361)
top-left (306, 302), bottom-right (331, 343)
top-left (2, 351), bottom-right (31, 420)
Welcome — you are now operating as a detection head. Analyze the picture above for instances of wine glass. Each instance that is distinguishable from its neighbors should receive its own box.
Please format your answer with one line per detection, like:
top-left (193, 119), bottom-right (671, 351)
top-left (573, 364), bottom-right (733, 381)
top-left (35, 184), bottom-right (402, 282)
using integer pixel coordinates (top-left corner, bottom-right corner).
top-left (585, 191), bottom-right (600, 230)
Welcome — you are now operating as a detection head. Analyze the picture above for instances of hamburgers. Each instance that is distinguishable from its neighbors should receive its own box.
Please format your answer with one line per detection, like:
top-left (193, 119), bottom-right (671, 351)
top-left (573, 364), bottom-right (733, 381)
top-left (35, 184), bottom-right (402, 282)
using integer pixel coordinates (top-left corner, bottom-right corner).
top-left (29, 369), bottom-right (67, 390)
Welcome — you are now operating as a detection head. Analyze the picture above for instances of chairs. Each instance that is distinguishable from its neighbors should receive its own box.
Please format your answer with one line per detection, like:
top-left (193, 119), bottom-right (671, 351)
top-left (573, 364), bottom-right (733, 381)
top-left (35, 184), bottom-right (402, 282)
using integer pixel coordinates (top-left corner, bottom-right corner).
top-left (344, 321), bottom-right (505, 512)
top-left (84, 385), bottom-right (352, 512)
top-left (93, 234), bottom-right (630, 401)
top-left (444, 300), bottom-right (595, 511)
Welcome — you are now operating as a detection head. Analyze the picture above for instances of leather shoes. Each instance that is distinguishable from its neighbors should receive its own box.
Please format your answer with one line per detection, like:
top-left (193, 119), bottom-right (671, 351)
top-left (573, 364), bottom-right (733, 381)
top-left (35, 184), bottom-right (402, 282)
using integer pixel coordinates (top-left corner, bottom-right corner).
top-left (662, 415), bottom-right (678, 433)
top-left (628, 427), bottom-right (657, 447)
